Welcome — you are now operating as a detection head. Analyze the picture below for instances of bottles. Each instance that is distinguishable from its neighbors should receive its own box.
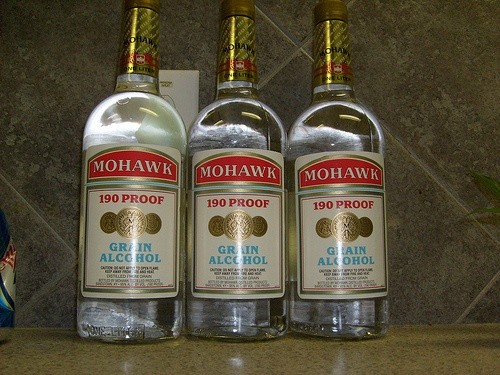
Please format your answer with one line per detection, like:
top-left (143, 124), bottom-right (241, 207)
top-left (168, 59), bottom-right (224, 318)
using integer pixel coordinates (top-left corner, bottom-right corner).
top-left (75, 0), bottom-right (186, 343)
top-left (286, 0), bottom-right (388, 342)
top-left (181, 1), bottom-right (292, 343)
